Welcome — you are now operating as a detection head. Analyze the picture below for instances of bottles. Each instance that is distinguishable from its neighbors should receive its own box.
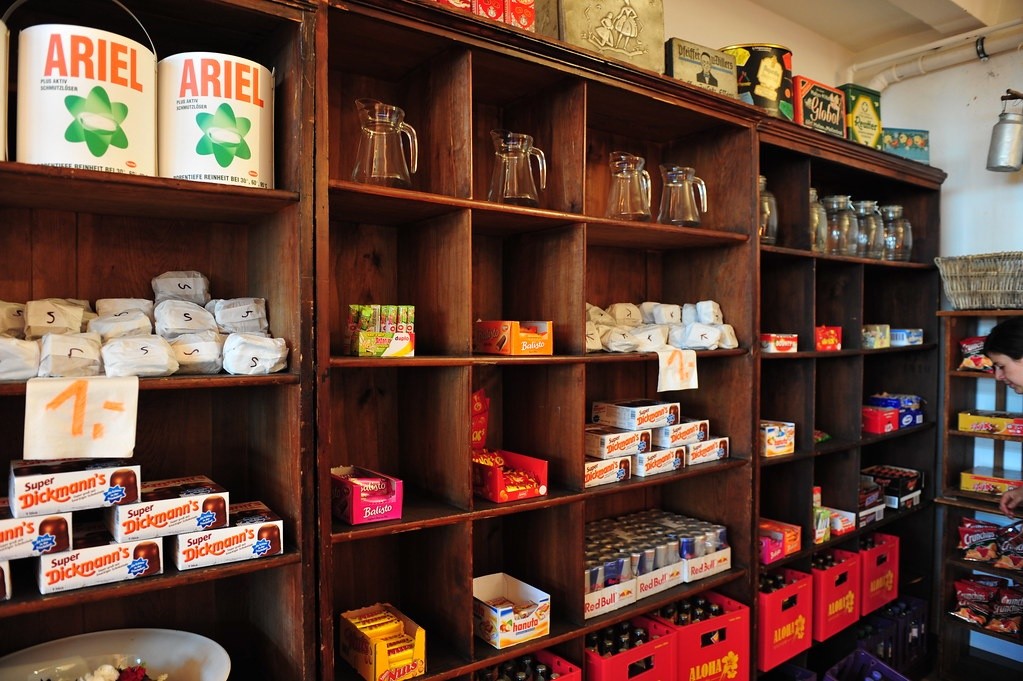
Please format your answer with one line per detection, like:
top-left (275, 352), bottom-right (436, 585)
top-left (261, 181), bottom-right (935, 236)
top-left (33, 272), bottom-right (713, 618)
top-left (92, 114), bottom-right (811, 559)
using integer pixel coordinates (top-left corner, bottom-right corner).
top-left (820, 194), bottom-right (858, 257)
top-left (584, 621), bottom-right (662, 680)
top-left (808, 187), bottom-right (828, 254)
top-left (655, 593), bottom-right (728, 650)
top-left (758, 174), bottom-right (777, 245)
top-left (751, 566), bottom-right (802, 611)
top-left (812, 536), bottom-right (890, 589)
top-left (473, 653), bottom-right (562, 681)
top-left (854, 200), bottom-right (885, 259)
top-left (880, 203), bottom-right (912, 261)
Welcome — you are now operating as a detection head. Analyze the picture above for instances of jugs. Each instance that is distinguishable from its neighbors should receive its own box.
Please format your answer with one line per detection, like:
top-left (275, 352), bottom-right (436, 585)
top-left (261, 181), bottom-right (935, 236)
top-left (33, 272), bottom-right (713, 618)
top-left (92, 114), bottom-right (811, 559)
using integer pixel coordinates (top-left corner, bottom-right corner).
top-left (352, 96), bottom-right (419, 190)
top-left (487, 128), bottom-right (547, 208)
top-left (659, 163), bottom-right (708, 228)
top-left (605, 149), bottom-right (653, 222)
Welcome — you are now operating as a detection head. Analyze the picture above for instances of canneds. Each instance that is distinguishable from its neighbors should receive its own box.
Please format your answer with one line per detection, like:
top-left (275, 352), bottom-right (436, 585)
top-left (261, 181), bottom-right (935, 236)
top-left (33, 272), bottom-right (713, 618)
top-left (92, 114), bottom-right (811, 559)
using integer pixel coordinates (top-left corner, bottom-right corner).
top-left (584, 510), bottom-right (726, 595)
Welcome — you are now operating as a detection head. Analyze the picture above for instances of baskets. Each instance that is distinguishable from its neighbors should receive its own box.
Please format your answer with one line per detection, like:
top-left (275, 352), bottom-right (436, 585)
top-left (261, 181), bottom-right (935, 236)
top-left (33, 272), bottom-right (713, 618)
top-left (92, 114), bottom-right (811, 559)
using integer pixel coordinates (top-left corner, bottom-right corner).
top-left (933, 251), bottom-right (1023, 310)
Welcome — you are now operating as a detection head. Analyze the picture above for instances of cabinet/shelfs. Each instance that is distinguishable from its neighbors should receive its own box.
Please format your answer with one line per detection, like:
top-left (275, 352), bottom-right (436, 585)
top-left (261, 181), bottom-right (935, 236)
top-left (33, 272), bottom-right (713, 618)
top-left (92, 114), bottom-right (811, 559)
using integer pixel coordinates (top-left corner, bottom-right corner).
top-left (754, 116), bottom-right (948, 681)
top-left (1, 1), bottom-right (319, 680)
top-left (931, 308), bottom-right (1023, 681)
top-left (317, 0), bottom-right (767, 681)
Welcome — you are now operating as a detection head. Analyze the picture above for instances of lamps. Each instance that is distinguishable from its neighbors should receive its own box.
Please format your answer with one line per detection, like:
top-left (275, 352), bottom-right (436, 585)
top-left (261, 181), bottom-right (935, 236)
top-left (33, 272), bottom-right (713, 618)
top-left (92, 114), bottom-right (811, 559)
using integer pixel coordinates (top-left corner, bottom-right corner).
top-left (986, 89), bottom-right (1023, 171)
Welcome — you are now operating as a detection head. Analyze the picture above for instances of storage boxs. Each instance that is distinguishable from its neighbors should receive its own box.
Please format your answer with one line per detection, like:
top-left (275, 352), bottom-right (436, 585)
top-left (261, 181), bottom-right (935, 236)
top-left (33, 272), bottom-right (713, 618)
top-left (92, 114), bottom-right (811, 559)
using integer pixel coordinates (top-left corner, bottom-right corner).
top-left (958, 410), bottom-right (1023, 436)
top-left (405, 0), bottom-right (929, 164)
top-left (960, 466), bottom-right (1023, 497)
top-left (1, 304), bottom-right (926, 681)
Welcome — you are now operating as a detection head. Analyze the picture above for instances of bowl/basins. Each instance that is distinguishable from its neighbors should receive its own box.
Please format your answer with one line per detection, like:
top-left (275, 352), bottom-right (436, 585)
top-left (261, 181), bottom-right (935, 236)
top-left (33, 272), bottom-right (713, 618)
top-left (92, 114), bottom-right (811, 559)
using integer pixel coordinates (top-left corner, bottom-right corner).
top-left (0, 628), bottom-right (232, 681)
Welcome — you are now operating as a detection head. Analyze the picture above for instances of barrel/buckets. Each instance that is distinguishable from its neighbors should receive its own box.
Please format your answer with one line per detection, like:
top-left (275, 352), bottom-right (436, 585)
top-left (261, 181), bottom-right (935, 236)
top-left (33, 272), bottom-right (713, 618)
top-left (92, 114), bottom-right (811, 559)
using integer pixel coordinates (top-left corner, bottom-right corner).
top-left (985, 90), bottom-right (1022, 174)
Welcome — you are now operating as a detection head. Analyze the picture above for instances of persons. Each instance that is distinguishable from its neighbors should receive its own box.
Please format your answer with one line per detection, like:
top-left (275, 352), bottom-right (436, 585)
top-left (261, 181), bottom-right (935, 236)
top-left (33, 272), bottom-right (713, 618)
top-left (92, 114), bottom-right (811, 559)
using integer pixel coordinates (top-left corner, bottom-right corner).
top-left (696, 52), bottom-right (718, 86)
top-left (983, 316), bottom-right (1023, 521)
